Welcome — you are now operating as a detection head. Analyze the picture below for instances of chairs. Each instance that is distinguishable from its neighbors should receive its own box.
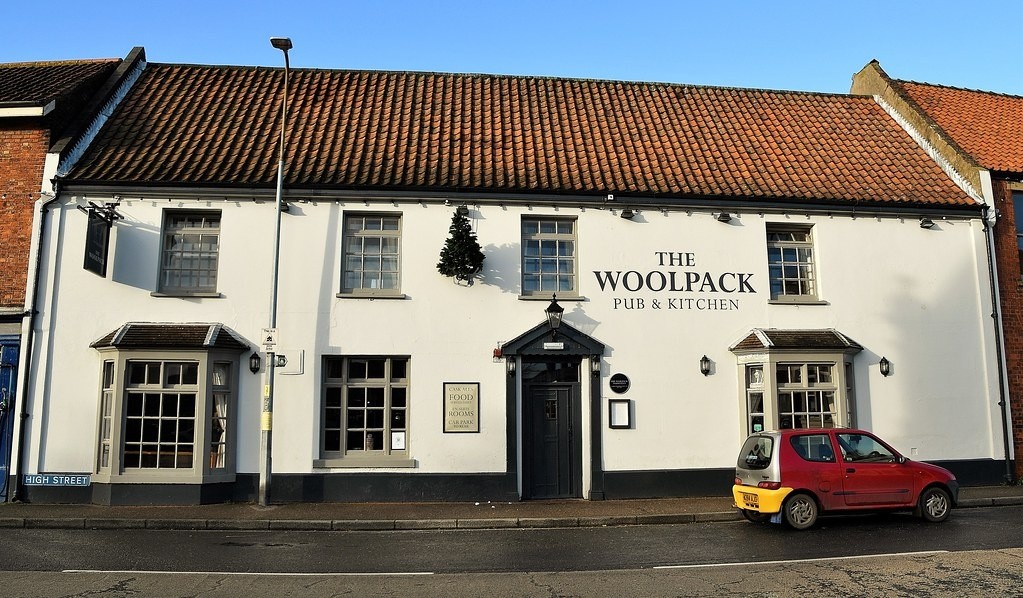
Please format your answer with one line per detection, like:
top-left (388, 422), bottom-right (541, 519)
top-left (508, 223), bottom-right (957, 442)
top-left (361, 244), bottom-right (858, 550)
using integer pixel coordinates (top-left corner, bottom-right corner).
top-left (819, 444), bottom-right (832, 460)
top-left (792, 437), bottom-right (806, 456)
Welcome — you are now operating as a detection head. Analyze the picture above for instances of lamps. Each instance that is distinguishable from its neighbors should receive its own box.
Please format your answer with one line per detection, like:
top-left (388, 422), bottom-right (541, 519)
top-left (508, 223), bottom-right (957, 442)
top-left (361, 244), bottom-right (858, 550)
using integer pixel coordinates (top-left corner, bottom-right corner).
top-left (621, 209), bottom-right (634, 218)
top-left (249, 352), bottom-right (261, 374)
top-left (275, 202), bottom-right (289, 212)
top-left (717, 214), bottom-right (732, 221)
top-left (700, 355), bottom-right (710, 376)
top-left (919, 216), bottom-right (935, 228)
top-left (456, 206), bottom-right (469, 215)
top-left (880, 357), bottom-right (889, 377)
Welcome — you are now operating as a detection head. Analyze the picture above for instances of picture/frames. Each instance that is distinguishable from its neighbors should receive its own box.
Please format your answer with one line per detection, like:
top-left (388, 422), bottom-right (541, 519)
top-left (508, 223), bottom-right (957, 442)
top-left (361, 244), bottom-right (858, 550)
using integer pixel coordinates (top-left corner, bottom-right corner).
top-left (608, 399), bottom-right (631, 430)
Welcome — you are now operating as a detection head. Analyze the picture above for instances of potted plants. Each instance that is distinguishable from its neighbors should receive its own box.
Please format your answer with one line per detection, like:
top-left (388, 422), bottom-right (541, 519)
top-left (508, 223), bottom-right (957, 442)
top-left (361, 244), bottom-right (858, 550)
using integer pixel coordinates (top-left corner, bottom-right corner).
top-left (438, 212), bottom-right (487, 286)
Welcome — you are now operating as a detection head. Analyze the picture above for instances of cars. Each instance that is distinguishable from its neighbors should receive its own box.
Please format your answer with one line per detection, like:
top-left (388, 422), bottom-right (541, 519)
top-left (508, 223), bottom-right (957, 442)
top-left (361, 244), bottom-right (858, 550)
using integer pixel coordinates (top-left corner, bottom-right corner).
top-left (731, 427), bottom-right (960, 532)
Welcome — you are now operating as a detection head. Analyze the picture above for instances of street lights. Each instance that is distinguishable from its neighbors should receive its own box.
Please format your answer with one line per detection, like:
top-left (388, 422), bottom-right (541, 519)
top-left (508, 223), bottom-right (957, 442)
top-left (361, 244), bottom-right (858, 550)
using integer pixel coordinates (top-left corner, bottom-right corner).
top-left (258, 36), bottom-right (295, 505)
